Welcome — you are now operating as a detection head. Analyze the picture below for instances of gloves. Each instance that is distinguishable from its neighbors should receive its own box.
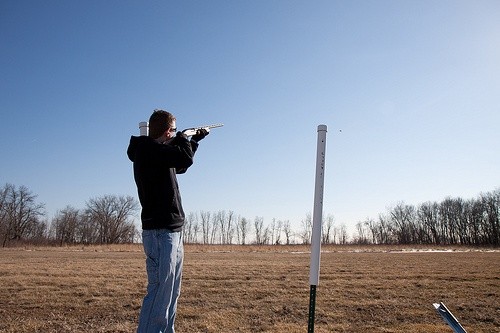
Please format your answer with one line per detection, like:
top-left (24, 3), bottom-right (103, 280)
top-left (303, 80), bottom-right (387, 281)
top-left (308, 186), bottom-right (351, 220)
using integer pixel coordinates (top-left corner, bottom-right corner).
top-left (176, 131), bottom-right (187, 140)
top-left (191, 129), bottom-right (209, 142)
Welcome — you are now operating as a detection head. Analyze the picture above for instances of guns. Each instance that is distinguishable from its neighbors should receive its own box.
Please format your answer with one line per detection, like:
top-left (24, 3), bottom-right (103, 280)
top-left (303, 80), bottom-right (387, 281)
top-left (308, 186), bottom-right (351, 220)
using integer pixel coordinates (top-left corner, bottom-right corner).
top-left (182, 122), bottom-right (224, 138)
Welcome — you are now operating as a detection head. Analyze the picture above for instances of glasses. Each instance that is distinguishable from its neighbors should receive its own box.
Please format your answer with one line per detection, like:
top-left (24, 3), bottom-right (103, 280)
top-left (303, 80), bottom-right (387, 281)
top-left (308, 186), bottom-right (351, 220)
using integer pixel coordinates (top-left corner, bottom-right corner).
top-left (170, 128), bottom-right (177, 132)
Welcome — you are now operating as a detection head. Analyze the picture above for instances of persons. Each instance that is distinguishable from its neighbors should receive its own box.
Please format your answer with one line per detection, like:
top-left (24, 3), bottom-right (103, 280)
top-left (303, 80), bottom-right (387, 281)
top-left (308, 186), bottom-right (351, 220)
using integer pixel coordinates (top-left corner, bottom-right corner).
top-left (133, 110), bottom-right (211, 333)
